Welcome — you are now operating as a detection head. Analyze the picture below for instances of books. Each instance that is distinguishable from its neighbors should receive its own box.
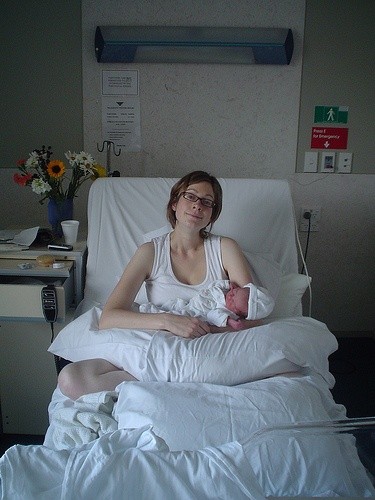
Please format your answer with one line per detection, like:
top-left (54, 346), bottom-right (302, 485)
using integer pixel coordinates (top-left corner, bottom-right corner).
top-left (0, 226), bottom-right (48, 251)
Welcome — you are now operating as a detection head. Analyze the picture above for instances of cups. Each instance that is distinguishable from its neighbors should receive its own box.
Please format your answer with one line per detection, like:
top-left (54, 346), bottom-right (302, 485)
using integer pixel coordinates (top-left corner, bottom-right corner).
top-left (61, 220), bottom-right (79, 244)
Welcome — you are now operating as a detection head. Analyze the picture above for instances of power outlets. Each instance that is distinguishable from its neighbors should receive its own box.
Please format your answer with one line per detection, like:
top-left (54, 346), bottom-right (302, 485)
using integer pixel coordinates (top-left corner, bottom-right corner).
top-left (338, 153), bottom-right (352, 173)
top-left (301, 206), bottom-right (321, 232)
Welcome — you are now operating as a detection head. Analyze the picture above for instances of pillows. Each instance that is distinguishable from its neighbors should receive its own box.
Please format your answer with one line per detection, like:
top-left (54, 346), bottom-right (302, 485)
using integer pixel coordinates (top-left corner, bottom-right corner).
top-left (144, 225), bottom-right (312, 324)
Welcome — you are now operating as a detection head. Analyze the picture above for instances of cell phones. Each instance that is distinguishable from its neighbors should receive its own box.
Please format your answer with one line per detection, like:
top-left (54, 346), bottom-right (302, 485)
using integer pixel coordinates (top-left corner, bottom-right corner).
top-left (48, 244), bottom-right (73, 251)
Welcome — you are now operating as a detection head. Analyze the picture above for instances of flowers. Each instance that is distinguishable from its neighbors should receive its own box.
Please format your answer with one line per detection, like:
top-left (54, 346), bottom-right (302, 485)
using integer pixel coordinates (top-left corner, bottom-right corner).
top-left (13, 145), bottom-right (106, 205)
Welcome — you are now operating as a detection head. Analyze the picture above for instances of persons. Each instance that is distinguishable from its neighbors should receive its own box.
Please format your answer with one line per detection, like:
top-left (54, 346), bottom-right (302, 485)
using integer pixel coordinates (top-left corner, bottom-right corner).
top-left (57, 171), bottom-right (265, 403)
top-left (139, 280), bottom-right (276, 330)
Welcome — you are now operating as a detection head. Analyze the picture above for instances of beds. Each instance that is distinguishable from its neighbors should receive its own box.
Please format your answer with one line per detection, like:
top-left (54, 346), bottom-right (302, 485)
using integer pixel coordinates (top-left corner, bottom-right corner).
top-left (0, 177), bottom-right (375, 500)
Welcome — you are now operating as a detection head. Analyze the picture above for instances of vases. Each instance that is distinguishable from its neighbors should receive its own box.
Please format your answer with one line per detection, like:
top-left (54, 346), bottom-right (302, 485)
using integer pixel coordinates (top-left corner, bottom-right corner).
top-left (48, 199), bottom-right (74, 237)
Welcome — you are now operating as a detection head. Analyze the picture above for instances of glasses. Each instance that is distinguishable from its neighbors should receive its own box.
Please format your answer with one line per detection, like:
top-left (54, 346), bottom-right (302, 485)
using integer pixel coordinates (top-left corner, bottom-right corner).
top-left (176, 192), bottom-right (216, 208)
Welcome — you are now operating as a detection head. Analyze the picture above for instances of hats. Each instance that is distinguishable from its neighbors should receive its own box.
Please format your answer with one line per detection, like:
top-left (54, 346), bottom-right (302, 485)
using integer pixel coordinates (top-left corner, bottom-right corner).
top-left (243, 283), bottom-right (275, 320)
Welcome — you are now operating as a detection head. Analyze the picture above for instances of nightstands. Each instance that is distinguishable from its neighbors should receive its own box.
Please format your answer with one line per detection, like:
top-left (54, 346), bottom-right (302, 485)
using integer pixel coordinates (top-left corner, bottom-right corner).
top-left (0, 231), bottom-right (89, 435)
